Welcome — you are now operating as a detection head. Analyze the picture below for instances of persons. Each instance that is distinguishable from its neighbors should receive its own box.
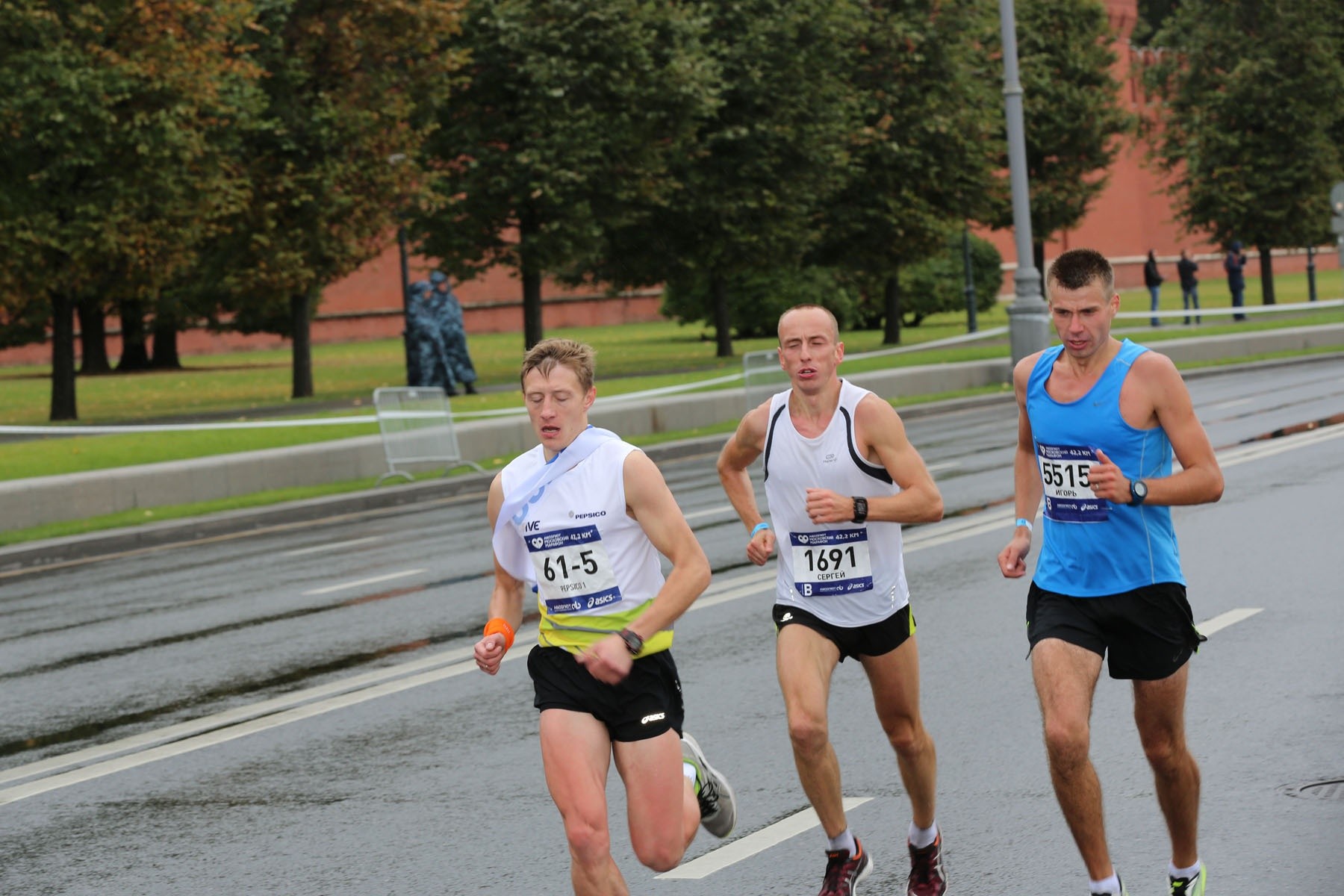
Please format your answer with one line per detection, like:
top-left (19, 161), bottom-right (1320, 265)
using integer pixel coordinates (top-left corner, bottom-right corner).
top-left (716, 305), bottom-right (946, 896)
top-left (996, 249), bottom-right (1224, 896)
top-left (1143, 250), bottom-right (1164, 327)
top-left (1224, 244), bottom-right (1246, 322)
top-left (1177, 249), bottom-right (1201, 325)
top-left (473, 338), bottom-right (737, 896)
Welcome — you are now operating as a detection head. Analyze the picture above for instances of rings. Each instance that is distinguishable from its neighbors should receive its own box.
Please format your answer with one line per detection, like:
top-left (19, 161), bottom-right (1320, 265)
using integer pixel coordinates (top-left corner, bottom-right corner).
top-left (486, 664), bottom-right (488, 669)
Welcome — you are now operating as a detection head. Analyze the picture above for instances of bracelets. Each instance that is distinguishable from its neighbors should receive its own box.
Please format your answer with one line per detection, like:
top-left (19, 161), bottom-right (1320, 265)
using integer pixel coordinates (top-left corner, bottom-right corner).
top-left (748, 520), bottom-right (770, 535)
top-left (1013, 519), bottom-right (1034, 532)
top-left (483, 617), bottom-right (515, 652)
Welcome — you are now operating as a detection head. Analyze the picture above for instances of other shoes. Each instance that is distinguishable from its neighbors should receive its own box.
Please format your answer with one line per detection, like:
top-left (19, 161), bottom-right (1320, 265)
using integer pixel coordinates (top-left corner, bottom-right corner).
top-left (1167, 861), bottom-right (1207, 896)
top-left (1088, 871), bottom-right (1130, 896)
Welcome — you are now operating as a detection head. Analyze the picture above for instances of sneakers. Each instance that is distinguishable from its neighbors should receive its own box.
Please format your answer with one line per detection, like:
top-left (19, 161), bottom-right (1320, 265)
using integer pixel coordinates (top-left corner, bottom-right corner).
top-left (816, 836), bottom-right (872, 896)
top-left (680, 731), bottom-right (736, 838)
top-left (905, 824), bottom-right (949, 896)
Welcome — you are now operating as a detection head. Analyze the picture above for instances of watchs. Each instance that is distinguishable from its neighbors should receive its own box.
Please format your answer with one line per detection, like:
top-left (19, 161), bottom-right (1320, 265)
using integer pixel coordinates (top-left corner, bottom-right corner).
top-left (852, 495), bottom-right (868, 524)
top-left (616, 628), bottom-right (644, 656)
top-left (1126, 472), bottom-right (1148, 509)
